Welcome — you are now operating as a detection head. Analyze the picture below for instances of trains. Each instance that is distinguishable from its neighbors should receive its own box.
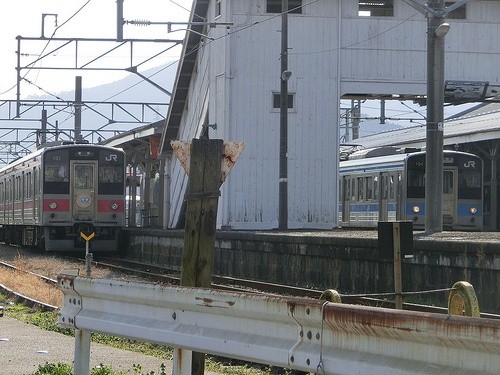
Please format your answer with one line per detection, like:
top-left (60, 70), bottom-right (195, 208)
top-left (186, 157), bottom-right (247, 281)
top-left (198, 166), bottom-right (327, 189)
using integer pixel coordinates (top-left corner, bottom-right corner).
top-left (336, 143), bottom-right (486, 233)
top-left (0, 135), bottom-right (127, 253)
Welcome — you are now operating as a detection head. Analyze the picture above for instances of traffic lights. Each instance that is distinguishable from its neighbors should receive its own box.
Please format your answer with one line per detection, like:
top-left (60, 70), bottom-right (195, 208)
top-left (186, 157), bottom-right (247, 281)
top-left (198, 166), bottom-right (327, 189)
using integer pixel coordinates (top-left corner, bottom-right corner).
top-left (80, 223), bottom-right (95, 242)
